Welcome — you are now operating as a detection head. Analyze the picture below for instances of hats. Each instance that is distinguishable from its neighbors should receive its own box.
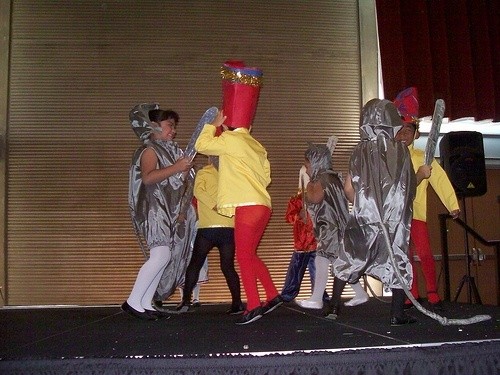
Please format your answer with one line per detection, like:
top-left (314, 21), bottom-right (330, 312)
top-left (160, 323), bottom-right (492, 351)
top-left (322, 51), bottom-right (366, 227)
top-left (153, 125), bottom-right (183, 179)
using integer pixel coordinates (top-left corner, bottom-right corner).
top-left (393, 87), bottom-right (420, 129)
top-left (220, 60), bottom-right (263, 128)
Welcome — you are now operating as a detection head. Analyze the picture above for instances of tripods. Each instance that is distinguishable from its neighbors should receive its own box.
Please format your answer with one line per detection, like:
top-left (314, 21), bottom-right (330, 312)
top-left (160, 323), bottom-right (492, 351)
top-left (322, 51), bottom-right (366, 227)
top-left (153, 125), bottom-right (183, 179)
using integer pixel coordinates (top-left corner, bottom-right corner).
top-left (453, 199), bottom-right (483, 305)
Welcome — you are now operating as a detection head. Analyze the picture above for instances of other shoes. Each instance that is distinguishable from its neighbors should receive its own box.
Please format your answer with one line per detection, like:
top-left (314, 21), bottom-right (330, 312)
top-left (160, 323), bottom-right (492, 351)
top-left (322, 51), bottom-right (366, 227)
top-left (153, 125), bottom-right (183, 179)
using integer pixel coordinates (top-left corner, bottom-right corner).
top-left (152, 298), bottom-right (163, 311)
top-left (121, 300), bottom-right (158, 321)
top-left (235, 306), bottom-right (262, 325)
top-left (144, 309), bottom-right (163, 318)
top-left (402, 296), bottom-right (422, 309)
top-left (296, 299), bottom-right (323, 309)
top-left (192, 302), bottom-right (201, 308)
top-left (176, 301), bottom-right (189, 312)
top-left (322, 304), bottom-right (338, 320)
top-left (262, 294), bottom-right (283, 314)
top-left (344, 293), bottom-right (369, 306)
top-left (390, 315), bottom-right (418, 327)
top-left (227, 305), bottom-right (245, 315)
top-left (427, 298), bottom-right (442, 307)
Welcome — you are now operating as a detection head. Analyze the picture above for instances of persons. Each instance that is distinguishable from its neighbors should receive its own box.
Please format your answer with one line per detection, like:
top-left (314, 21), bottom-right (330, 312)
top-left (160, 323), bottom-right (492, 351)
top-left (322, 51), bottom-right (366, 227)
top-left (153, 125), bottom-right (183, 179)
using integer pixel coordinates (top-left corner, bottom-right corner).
top-left (280, 163), bottom-right (331, 307)
top-left (391, 102), bottom-right (461, 307)
top-left (193, 110), bottom-right (284, 326)
top-left (175, 155), bottom-right (245, 317)
top-left (119, 102), bottom-right (195, 323)
top-left (294, 144), bottom-right (370, 310)
top-left (321, 98), bottom-right (423, 327)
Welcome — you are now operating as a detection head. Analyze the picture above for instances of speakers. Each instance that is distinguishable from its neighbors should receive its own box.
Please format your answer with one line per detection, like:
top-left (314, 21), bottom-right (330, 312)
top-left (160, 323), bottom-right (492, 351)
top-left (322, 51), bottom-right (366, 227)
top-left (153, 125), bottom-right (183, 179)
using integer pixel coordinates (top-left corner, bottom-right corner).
top-left (439, 131), bottom-right (487, 198)
